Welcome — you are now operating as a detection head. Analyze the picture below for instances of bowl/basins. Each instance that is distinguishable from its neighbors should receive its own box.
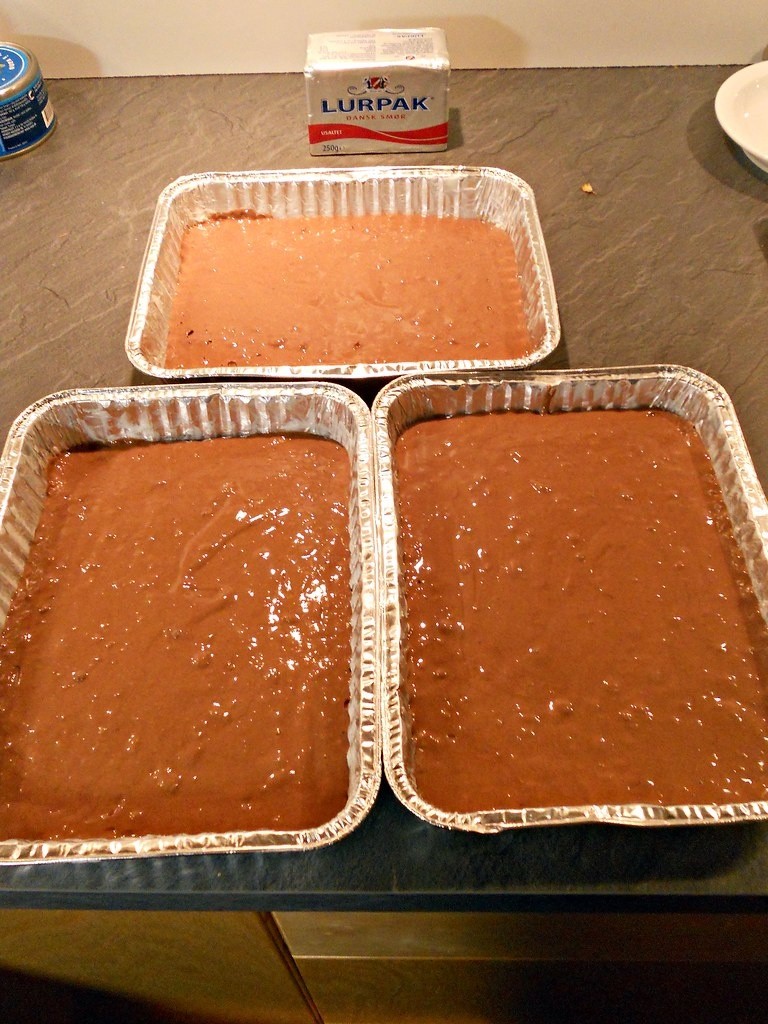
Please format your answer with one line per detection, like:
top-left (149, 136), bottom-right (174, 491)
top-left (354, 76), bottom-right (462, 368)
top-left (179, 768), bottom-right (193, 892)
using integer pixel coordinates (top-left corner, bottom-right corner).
top-left (0, 380), bottom-right (382, 864)
top-left (125, 166), bottom-right (562, 377)
top-left (714, 60), bottom-right (768, 172)
top-left (372, 364), bottom-right (768, 838)
